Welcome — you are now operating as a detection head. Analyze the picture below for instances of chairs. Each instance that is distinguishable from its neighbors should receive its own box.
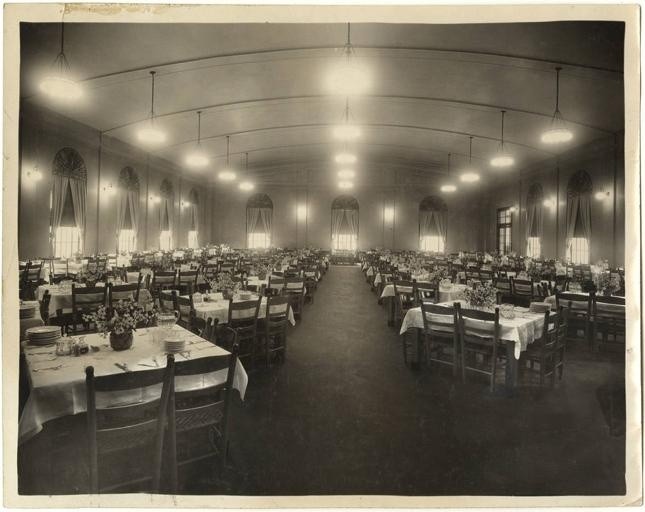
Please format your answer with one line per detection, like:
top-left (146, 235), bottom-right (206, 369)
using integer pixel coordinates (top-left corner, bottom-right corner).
top-left (353, 251), bottom-right (405, 277)
top-left (158, 288), bottom-right (177, 310)
top-left (517, 300), bottom-right (573, 388)
top-left (591, 291), bottom-right (625, 353)
top-left (222, 295), bottom-right (262, 370)
top-left (212, 244), bottom-right (282, 269)
top-left (420, 299), bottom-right (458, 378)
top-left (38, 290), bottom-right (52, 321)
top-left (65, 282), bottom-right (108, 335)
top-left (419, 251), bottom-right (543, 292)
top-left (152, 269), bottom-right (176, 292)
top-left (90, 253), bottom-right (116, 277)
top-left (556, 288), bottom-right (592, 353)
top-left (457, 302), bottom-right (507, 393)
top-left (415, 279), bottom-right (439, 306)
top-left (21, 257), bottom-right (41, 278)
top-left (40, 289), bottom-right (52, 321)
top-left (167, 343), bottom-right (239, 491)
top-left (546, 256), bottom-right (597, 283)
top-left (109, 283), bottom-right (140, 318)
top-left (175, 291), bottom-right (206, 330)
top-left (269, 279), bottom-right (305, 323)
top-left (47, 353), bottom-right (174, 495)
top-left (283, 243), bottom-right (340, 275)
top-left (393, 278), bottom-right (417, 331)
top-left (191, 317), bottom-right (237, 355)
top-left (178, 267), bottom-right (200, 291)
top-left (256, 296), bottom-right (292, 369)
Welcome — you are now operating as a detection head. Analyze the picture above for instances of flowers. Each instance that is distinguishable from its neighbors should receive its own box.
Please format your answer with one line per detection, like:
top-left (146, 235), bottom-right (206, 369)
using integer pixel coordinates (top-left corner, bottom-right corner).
top-left (82, 298), bottom-right (145, 339)
top-left (596, 272), bottom-right (621, 292)
top-left (462, 286), bottom-right (497, 305)
top-left (210, 271), bottom-right (241, 289)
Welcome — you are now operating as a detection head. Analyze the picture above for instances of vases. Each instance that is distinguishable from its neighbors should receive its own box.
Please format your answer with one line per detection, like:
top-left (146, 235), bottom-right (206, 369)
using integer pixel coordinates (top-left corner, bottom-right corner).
top-left (222, 288), bottom-right (234, 299)
top-left (109, 330), bottom-right (133, 351)
top-left (471, 302), bottom-right (489, 312)
top-left (603, 289), bottom-right (612, 297)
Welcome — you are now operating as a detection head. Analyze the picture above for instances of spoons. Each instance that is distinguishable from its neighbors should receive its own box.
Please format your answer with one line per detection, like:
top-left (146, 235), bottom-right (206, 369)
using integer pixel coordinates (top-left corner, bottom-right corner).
top-left (28, 352), bottom-right (52, 355)
top-left (152, 356), bottom-right (159, 367)
top-left (185, 340), bottom-right (207, 345)
top-left (33, 364), bottom-right (62, 372)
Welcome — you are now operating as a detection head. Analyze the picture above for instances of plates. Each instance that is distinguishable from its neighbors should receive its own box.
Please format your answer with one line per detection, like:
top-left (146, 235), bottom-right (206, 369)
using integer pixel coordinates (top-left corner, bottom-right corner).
top-left (25, 325), bottom-right (62, 345)
top-left (20, 306), bottom-right (35, 319)
top-left (529, 302), bottom-right (552, 313)
top-left (161, 289), bottom-right (179, 296)
top-left (240, 292), bottom-right (251, 300)
top-left (164, 337), bottom-right (185, 352)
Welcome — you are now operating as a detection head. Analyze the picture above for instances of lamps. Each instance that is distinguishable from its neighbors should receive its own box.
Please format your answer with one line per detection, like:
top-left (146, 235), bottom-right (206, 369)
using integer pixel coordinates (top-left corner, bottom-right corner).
top-left (461, 137), bottom-right (480, 183)
top-left (330, 22), bottom-right (371, 94)
top-left (333, 97), bottom-right (361, 135)
top-left (40, 22), bottom-right (82, 101)
top-left (489, 110), bottom-right (515, 167)
top-left (238, 152), bottom-right (254, 191)
top-left (440, 154), bottom-right (456, 193)
top-left (217, 136), bottom-right (236, 180)
top-left (186, 110), bottom-right (209, 168)
top-left (335, 140), bottom-right (357, 164)
top-left (138, 71), bottom-right (165, 143)
top-left (542, 68), bottom-right (573, 144)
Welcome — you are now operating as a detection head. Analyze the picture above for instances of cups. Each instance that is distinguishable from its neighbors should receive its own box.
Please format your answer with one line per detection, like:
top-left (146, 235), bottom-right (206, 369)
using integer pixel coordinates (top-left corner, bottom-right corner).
top-left (500, 303), bottom-right (516, 320)
top-left (158, 309), bottom-right (181, 331)
